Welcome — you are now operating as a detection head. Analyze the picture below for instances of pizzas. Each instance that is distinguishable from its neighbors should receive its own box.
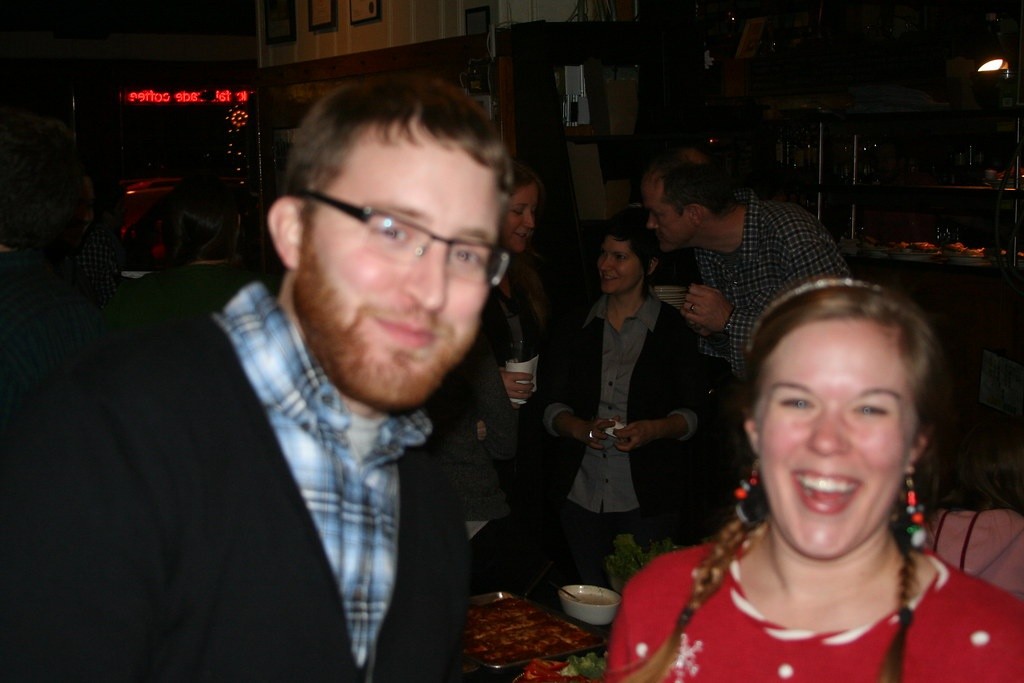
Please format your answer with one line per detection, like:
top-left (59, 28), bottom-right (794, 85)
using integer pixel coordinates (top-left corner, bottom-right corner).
top-left (461, 597), bottom-right (604, 665)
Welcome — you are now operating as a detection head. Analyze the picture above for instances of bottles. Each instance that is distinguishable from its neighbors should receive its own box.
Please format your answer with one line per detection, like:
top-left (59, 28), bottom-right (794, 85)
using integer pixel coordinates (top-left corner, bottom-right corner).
top-left (776, 126), bottom-right (819, 172)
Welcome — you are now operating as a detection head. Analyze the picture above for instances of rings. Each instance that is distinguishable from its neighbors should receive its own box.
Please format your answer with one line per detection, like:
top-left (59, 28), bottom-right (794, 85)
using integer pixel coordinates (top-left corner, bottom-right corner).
top-left (589, 430), bottom-right (593, 438)
top-left (689, 304), bottom-right (695, 310)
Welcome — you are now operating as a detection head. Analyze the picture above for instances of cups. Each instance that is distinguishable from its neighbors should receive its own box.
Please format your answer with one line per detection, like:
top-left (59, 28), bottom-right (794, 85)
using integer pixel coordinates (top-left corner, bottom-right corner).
top-left (510, 336), bottom-right (535, 363)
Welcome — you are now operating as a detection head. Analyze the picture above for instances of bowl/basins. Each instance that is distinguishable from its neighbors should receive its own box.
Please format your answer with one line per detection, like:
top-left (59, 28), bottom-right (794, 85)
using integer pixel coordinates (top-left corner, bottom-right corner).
top-left (558, 584), bottom-right (622, 625)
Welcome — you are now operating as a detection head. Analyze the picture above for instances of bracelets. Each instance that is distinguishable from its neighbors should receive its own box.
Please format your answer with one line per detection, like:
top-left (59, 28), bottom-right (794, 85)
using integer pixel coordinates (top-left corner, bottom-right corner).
top-left (723, 308), bottom-right (736, 332)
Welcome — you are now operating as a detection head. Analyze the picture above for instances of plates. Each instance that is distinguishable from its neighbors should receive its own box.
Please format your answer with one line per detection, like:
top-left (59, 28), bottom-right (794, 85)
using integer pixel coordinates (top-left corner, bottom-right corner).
top-left (863, 248), bottom-right (889, 258)
top-left (888, 254), bottom-right (935, 262)
top-left (936, 256), bottom-right (984, 263)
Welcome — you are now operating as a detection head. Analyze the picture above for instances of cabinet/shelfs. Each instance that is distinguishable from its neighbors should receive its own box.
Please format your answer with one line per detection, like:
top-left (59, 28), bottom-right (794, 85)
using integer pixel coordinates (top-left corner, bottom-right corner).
top-left (788, 105), bottom-right (1023, 279)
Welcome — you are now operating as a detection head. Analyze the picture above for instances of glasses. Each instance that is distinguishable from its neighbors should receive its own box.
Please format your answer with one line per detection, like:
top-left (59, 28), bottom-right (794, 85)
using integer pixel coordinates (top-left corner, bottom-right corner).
top-left (294, 188), bottom-right (509, 287)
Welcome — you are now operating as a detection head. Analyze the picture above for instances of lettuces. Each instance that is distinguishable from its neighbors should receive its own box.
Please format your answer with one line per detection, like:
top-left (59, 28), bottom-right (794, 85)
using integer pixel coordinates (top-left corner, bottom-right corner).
top-left (556, 651), bottom-right (607, 681)
top-left (604, 532), bottom-right (695, 583)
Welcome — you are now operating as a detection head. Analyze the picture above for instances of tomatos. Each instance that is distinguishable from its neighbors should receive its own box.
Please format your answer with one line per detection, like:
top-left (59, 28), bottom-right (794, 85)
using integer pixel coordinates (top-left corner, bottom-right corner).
top-left (520, 657), bottom-right (590, 683)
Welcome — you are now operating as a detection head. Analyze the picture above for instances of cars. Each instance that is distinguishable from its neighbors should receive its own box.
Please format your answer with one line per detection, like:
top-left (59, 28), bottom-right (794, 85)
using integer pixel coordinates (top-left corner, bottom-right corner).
top-left (119, 176), bottom-right (245, 227)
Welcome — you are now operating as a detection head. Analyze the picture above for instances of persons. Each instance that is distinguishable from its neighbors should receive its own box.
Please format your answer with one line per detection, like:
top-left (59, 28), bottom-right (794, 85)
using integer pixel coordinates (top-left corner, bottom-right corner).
top-left (0, 70), bottom-right (517, 683)
top-left (595, 276), bottom-right (1024, 683)
top-left (0, 117), bottom-right (858, 604)
